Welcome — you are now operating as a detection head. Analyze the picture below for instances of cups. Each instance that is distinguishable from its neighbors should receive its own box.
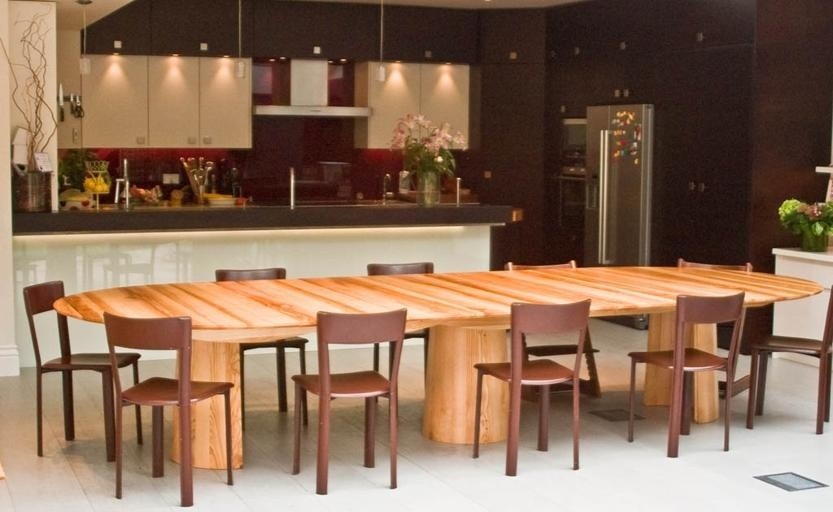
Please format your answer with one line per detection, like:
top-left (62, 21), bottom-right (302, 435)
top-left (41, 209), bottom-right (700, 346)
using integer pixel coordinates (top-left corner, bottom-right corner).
top-left (398, 171), bottom-right (410, 193)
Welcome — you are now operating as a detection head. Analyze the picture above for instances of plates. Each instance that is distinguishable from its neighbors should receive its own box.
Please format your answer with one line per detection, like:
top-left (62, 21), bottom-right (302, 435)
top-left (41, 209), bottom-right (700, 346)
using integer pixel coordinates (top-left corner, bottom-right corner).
top-left (207, 194), bottom-right (247, 206)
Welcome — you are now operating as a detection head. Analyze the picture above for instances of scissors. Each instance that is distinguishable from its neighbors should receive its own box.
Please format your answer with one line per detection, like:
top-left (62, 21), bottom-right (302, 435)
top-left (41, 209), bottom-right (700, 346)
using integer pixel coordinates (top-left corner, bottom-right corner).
top-left (74, 96), bottom-right (84, 118)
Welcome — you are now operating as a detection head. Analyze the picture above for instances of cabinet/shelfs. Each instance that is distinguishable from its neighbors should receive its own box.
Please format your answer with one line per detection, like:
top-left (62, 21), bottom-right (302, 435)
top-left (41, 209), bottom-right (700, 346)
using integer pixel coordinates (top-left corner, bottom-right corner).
top-left (56, 28), bottom-right (81, 148)
top-left (351, 59), bottom-right (472, 150)
top-left (150, 54), bottom-right (254, 150)
top-left (81, 54), bottom-right (150, 150)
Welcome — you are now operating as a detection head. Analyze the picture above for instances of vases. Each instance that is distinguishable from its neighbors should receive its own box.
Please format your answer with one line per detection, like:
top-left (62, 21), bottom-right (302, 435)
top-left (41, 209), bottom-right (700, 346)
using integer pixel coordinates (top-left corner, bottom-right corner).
top-left (797, 221), bottom-right (830, 253)
top-left (415, 154), bottom-right (442, 209)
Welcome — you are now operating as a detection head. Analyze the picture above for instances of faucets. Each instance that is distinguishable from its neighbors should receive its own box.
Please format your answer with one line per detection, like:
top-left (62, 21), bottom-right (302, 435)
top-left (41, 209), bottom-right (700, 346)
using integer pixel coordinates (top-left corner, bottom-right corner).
top-left (382, 174), bottom-right (391, 204)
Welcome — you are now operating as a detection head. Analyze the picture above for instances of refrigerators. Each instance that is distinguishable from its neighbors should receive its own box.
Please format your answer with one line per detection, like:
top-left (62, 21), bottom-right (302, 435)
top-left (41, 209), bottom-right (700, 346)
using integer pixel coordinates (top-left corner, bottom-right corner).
top-left (583, 103), bottom-right (677, 330)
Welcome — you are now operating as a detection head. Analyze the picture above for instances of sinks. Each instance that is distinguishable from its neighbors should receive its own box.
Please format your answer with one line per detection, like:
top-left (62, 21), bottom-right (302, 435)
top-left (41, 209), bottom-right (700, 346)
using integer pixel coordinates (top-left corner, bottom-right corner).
top-left (287, 200), bottom-right (377, 206)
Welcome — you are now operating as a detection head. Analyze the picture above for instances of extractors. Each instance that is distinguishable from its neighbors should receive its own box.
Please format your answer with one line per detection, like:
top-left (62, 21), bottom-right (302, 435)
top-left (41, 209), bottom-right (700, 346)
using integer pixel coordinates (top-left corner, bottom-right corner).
top-left (252, 60), bottom-right (370, 116)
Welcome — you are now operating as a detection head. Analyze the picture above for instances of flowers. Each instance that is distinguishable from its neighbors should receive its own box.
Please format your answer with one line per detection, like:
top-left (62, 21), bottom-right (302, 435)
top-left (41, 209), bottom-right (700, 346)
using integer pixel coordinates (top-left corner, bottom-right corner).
top-left (779, 197), bottom-right (833, 237)
top-left (388, 107), bottom-right (469, 187)
